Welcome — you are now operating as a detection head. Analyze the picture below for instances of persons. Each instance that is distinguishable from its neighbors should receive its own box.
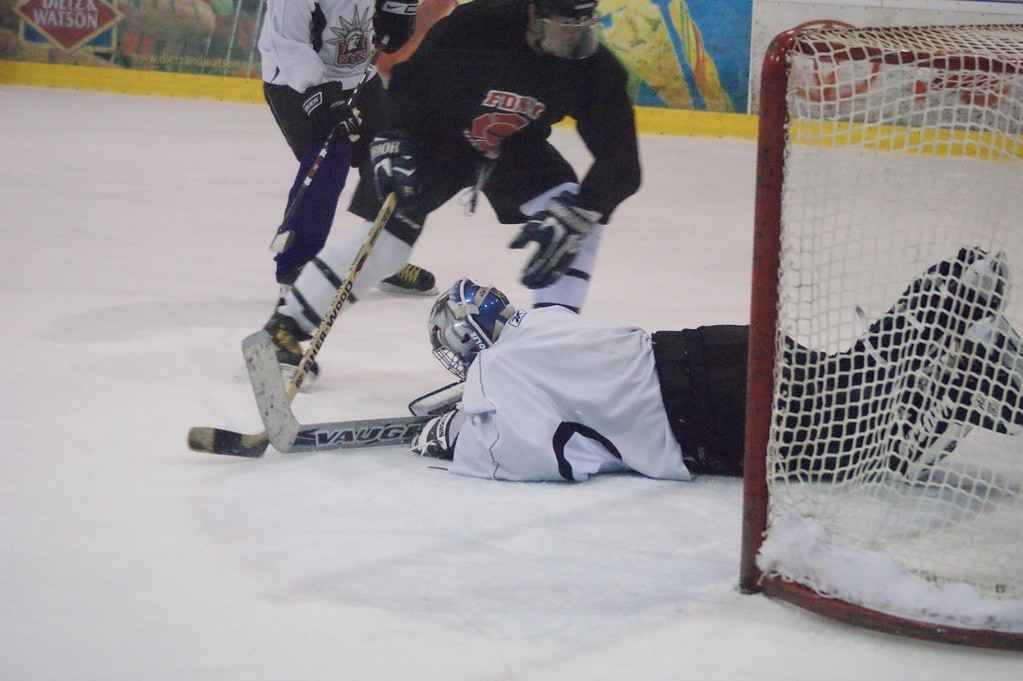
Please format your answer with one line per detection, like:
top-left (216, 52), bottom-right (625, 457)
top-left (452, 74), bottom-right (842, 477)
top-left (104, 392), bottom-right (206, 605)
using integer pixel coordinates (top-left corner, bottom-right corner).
top-left (411, 245), bottom-right (1023, 483)
top-left (258, 0), bottom-right (643, 393)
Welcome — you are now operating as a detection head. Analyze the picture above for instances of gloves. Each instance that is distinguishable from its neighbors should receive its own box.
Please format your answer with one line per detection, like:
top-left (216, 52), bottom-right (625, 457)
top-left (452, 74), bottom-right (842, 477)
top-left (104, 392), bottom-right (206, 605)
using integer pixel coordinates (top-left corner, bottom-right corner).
top-left (411, 410), bottom-right (456, 461)
top-left (509, 191), bottom-right (603, 289)
top-left (303, 81), bottom-right (360, 149)
top-left (373, 0), bottom-right (418, 55)
top-left (370, 130), bottom-right (420, 216)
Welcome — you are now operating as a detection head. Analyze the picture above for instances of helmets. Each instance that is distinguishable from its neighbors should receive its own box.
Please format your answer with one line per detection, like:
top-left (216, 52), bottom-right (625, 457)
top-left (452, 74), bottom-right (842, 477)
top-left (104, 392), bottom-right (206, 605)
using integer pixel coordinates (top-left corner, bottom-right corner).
top-left (515, 0), bottom-right (598, 20)
top-left (426, 276), bottom-right (513, 384)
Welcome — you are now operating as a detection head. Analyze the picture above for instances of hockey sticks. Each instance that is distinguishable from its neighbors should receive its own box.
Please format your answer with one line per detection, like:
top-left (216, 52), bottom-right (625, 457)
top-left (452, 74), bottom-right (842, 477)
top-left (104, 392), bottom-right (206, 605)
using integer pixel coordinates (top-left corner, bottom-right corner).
top-left (237, 324), bottom-right (434, 455)
top-left (184, 189), bottom-right (401, 460)
top-left (267, 33), bottom-right (393, 255)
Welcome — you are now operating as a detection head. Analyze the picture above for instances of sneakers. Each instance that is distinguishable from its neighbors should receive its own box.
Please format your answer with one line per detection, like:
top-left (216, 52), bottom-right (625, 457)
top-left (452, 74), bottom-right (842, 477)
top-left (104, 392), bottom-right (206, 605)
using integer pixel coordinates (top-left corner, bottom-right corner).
top-left (376, 261), bottom-right (440, 297)
top-left (263, 298), bottom-right (319, 391)
